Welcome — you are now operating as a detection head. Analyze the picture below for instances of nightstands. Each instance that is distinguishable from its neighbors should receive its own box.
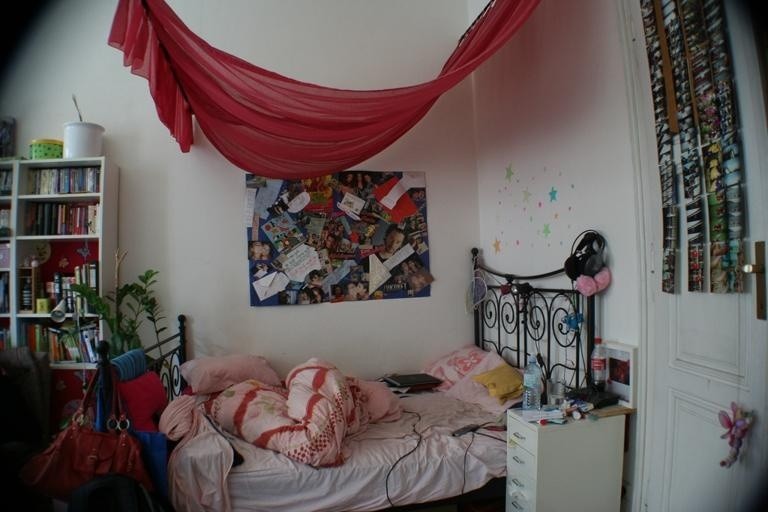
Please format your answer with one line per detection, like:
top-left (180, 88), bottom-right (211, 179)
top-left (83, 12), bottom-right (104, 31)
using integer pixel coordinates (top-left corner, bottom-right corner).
top-left (505, 408), bottom-right (626, 511)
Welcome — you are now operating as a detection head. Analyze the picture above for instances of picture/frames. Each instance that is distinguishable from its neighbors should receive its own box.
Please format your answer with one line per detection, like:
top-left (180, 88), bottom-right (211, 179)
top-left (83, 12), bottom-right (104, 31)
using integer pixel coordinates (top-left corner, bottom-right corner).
top-left (605, 340), bottom-right (638, 410)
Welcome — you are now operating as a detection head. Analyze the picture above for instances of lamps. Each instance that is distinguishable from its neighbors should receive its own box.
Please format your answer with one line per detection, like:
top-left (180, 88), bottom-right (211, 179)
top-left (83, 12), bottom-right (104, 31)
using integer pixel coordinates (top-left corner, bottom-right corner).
top-left (48, 295), bottom-right (73, 323)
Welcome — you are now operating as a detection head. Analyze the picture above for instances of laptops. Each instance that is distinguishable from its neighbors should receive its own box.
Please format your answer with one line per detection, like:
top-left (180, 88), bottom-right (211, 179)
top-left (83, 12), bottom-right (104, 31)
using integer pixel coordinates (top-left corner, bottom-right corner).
top-left (383, 373), bottom-right (443, 387)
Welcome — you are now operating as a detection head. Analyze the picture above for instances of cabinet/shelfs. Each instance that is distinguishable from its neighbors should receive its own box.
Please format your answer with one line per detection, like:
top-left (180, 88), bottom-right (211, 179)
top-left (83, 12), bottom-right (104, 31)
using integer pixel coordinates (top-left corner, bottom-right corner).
top-left (0, 157), bottom-right (120, 370)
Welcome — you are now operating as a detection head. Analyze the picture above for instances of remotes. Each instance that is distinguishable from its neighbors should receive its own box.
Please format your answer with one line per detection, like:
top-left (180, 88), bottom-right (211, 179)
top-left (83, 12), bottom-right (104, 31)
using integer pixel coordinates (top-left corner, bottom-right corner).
top-left (453, 423), bottom-right (480, 438)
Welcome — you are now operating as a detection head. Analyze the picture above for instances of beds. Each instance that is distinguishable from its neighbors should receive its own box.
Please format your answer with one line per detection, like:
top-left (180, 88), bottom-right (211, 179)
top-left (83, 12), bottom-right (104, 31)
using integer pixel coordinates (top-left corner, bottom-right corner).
top-left (94, 232), bottom-right (599, 511)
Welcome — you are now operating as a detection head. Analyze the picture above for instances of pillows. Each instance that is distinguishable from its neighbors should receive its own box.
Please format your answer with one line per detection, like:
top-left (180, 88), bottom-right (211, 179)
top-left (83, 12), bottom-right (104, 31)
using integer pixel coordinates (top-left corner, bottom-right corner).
top-left (175, 352), bottom-right (282, 396)
top-left (426, 345), bottom-right (526, 415)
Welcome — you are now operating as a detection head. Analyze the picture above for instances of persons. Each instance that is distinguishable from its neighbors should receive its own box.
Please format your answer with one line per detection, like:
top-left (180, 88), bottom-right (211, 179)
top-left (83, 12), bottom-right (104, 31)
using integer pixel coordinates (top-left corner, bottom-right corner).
top-left (247, 173), bottom-right (433, 303)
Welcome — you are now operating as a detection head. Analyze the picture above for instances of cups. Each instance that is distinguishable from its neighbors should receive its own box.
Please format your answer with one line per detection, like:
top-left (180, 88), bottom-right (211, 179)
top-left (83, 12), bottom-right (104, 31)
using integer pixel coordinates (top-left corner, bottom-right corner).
top-left (547, 378), bottom-right (567, 406)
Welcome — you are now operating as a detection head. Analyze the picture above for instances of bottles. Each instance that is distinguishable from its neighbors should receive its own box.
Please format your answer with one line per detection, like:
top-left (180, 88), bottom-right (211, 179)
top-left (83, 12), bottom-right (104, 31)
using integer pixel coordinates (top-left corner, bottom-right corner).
top-left (522, 355), bottom-right (542, 409)
top-left (589, 337), bottom-right (606, 388)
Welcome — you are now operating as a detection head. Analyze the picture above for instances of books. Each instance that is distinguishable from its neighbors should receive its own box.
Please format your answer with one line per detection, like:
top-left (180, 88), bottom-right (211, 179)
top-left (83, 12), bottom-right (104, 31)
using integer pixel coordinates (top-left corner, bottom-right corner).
top-left (0, 167), bottom-right (100, 363)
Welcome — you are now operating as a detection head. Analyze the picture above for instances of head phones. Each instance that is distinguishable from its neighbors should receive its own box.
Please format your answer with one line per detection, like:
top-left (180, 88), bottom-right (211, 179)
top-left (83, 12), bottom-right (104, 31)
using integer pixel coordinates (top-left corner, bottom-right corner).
top-left (564, 229), bottom-right (606, 281)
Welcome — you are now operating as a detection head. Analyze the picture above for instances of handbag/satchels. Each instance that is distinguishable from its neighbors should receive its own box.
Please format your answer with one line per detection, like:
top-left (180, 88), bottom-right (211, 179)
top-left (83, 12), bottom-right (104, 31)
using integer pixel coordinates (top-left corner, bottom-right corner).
top-left (18, 364), bottom-right (155, 503)
